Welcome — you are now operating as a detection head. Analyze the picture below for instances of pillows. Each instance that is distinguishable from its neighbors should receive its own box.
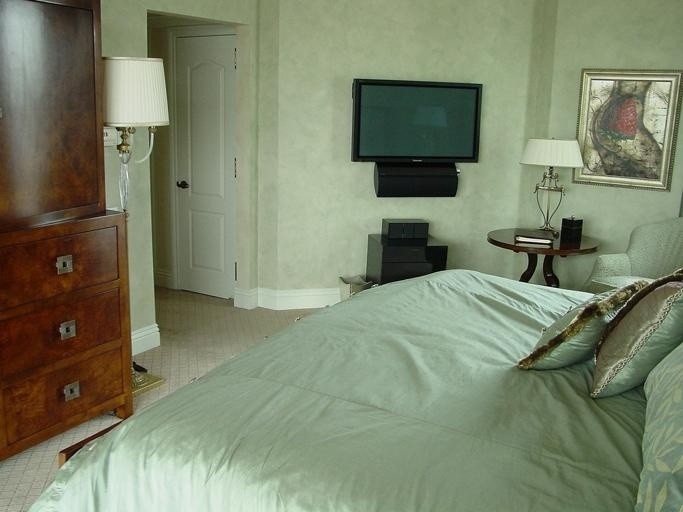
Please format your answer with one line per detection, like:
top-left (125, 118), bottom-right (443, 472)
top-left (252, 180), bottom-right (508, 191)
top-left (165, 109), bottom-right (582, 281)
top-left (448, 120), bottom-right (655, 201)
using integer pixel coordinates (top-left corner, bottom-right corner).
top-left (514, 280), bottom-right (649, 371)
top-left (588, 274), bottom-right (682, 398)
top-left (634, 340), bottom-right (682, 512)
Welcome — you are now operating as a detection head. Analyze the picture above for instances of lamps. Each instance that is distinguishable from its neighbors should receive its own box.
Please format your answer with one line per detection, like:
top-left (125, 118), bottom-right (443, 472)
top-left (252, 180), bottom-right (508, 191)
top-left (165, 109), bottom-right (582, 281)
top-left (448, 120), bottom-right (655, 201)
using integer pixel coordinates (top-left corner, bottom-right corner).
top-left (103, 57), bottom-right (171, 396)
top-left (519, 137), bottom-right (584, 228)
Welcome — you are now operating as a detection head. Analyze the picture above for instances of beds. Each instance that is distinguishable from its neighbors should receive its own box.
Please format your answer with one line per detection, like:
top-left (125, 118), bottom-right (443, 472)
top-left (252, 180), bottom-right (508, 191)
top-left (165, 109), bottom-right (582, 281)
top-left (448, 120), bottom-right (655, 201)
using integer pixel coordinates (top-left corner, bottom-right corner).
top-left (25, 266), bottom-right (643, 512)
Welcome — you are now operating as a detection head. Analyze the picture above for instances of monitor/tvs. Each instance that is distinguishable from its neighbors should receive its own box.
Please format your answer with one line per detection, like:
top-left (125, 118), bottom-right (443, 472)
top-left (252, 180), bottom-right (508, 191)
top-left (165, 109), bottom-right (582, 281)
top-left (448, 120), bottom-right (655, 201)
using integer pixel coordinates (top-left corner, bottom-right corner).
top-left (350, 78), bottom-right (482, 163)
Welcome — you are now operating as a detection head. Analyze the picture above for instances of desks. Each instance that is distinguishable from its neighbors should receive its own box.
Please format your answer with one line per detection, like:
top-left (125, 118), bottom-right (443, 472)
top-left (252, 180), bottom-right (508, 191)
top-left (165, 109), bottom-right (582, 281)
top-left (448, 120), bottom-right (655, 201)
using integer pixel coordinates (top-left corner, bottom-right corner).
top-left (485, 227), bottom-right (601, 289)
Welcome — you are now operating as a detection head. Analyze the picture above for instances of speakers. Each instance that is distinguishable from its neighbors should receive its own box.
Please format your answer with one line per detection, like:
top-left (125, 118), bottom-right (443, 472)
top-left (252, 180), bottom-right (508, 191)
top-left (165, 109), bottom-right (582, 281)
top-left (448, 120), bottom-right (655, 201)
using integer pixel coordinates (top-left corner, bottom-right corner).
top-left (372, 162), bottom-right (458, 198)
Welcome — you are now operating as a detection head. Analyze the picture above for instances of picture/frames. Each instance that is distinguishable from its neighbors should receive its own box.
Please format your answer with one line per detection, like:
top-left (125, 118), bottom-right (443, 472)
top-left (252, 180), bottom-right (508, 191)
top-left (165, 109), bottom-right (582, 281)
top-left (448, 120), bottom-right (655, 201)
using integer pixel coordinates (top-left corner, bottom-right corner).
top-left (572, 68), bottom-right (682, 194)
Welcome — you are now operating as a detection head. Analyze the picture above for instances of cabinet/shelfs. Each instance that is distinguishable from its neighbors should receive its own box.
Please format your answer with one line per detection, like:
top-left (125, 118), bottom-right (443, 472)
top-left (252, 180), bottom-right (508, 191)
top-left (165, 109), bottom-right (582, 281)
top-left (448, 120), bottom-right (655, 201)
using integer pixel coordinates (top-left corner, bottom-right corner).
top-left (0, 0), bottom-right (107, 229)
top-left (0, 208), bottom-right (134, 461)
top-left (365, 233), bottom-right (448, 289)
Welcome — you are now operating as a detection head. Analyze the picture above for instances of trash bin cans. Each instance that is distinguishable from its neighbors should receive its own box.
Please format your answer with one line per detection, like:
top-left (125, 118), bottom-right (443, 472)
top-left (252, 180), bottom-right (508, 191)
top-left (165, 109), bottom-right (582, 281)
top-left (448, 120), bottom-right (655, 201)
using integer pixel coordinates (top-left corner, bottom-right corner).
top-left (338, 274), bottom-right (373, 301)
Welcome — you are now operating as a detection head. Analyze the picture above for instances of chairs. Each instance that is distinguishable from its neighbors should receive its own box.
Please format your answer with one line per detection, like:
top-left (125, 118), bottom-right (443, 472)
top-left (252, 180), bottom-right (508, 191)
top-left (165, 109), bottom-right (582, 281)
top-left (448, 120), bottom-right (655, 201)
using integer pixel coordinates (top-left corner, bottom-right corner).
top-left (580, 217), bottom-right (682, 293)
